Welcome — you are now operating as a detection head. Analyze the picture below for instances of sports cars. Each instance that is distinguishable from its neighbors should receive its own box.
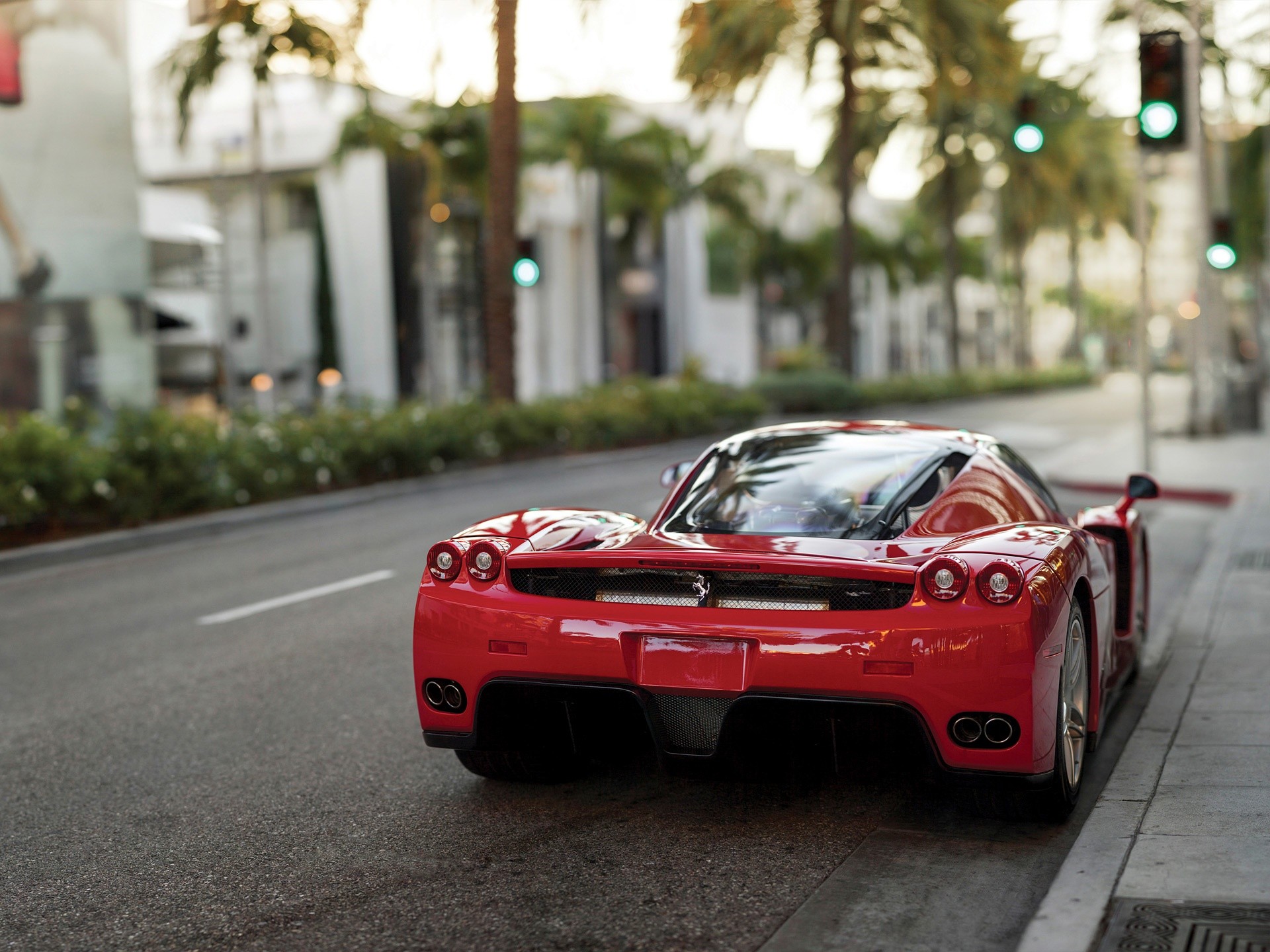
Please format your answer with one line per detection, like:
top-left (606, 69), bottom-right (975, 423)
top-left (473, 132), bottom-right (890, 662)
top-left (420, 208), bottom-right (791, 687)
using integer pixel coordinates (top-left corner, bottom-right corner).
top-left (413, 420), bottom-right (1162, 825)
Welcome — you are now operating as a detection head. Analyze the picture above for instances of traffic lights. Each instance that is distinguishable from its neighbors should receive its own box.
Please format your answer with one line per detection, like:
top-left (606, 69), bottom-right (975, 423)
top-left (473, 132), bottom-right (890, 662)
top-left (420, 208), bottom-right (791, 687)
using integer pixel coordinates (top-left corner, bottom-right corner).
top-left (1013, 95), bottom-right (1043, 163)
top-left (1208, 218), bottom-right (1240, 271)
top-left (1137, 29), bottom-right (1187, 152)
top-left (512, 238), bottom-right (539, 292)
